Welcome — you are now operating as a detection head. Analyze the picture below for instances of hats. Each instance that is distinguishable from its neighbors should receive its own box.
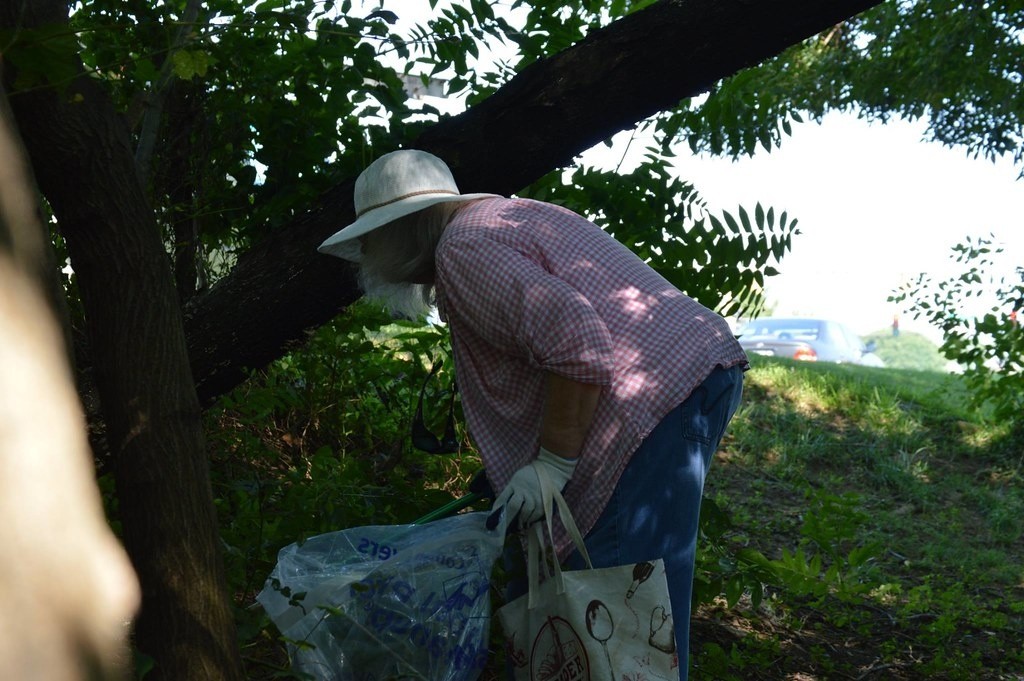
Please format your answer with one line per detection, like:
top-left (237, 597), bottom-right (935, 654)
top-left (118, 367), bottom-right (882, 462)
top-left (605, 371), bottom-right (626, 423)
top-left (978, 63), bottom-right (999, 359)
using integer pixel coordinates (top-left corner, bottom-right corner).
top-left (316, 149), bottom-right (503, 261)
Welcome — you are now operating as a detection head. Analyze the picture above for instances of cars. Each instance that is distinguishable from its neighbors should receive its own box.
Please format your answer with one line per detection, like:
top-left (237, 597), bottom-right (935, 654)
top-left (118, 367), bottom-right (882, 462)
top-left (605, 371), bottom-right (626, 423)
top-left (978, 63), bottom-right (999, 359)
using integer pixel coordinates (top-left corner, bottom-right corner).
top-left (740, 317), bottom-right (886, 366)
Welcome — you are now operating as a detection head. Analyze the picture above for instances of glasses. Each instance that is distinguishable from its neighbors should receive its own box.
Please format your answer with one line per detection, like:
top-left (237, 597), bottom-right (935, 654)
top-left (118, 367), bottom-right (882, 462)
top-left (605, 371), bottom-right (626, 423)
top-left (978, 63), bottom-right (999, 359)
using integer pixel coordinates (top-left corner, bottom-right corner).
top-left (412, 361), bottom-right (459, 454)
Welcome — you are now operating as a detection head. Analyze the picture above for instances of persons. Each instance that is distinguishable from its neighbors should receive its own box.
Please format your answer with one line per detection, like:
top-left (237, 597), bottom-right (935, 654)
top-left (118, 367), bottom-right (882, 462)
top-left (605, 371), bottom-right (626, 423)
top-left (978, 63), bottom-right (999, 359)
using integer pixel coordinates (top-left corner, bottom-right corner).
top-left (317, 148), bottom-right (750, 681)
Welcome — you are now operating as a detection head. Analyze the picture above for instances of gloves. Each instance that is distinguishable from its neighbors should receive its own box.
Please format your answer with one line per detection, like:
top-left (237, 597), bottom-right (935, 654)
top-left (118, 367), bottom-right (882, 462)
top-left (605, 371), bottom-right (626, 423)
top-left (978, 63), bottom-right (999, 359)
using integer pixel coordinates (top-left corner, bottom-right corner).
top-left (492, 446), bottom-right (580, 530)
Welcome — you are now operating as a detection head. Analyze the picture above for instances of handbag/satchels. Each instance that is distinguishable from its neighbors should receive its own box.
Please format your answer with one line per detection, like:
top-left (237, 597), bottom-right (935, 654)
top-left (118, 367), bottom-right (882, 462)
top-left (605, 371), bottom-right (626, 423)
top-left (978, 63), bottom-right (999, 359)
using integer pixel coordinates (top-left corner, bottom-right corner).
top-left (253, 510), bottom-right (504, 681)
top-left (495, 459), bottom-right (680, 681)
